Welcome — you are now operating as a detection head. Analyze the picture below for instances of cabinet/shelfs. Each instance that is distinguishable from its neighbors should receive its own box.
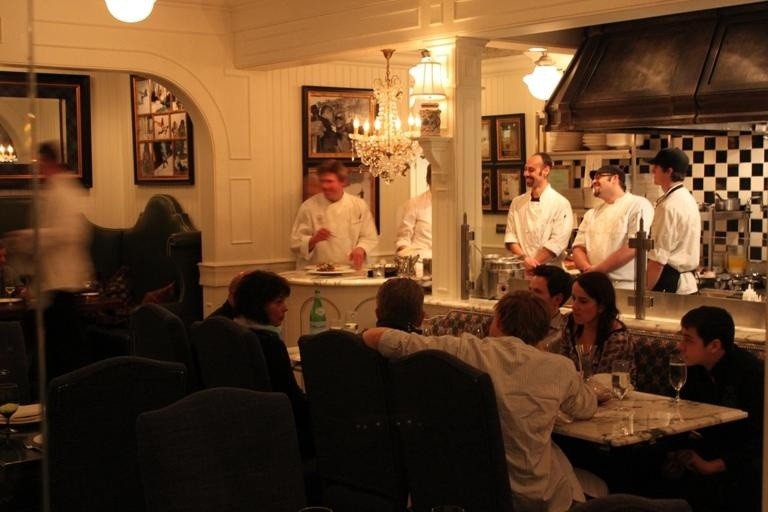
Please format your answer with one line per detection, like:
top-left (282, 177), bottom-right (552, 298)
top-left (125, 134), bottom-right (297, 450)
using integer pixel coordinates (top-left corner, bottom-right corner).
top-left (535, 112), bottom-right (750, 273)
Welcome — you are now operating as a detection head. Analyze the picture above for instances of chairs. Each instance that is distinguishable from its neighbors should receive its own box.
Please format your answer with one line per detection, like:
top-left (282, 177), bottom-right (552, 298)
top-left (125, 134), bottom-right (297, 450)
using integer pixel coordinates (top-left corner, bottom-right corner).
top-left (302, 331), bottom-right (517, 507)
top-left (38, 358), bottom-right (301, 510)
top-left (131, 300), bottom-right (272, 389)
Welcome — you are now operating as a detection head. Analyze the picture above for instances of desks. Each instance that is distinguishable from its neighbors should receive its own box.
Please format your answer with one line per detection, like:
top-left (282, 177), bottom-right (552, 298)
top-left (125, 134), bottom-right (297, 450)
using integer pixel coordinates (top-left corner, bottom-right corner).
top-left (274, 264), bottom-right (429, 317)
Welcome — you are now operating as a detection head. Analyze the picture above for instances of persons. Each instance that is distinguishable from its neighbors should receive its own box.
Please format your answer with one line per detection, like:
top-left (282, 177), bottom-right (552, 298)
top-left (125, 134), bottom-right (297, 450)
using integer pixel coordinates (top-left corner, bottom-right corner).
top-left (311, 103), bottom-right (363, 153)
top-left (133, 88), bottom-right (188, 177)
top-left (291, 159), bottom-right (378, 269)
top-left (572, 166), bottom-right (655, 290)
top-left (504, 153), bottom-right (573, 271)
top-left (644, 147), bottom-right (701, 293)
top-left (528, 263), bottom-right (572, 352)
top-left (483, 176), bottom-right (492, 204)
top-left (372, 279), bottom-right (425, 343)
top-left (667, 307), bottom-right (762, 512)
top-left (396, 164), bottom-right (433, 262)
top-left (13, 141), bottom-right (91, 369)
top-left (560, 271), bottom-right (636, 389)
top-left (357, 291), bottom-right (611, 510)
top-left (205, 270), bottom-right (308, 416)
top-left (204, 271), bottom-right (247, 320)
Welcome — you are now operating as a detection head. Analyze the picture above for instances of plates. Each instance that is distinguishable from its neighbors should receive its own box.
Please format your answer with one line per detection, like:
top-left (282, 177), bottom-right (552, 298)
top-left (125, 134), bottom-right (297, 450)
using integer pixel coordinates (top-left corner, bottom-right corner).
top-left (544, 131), bottom-right (643, 152)
top-left (303, 264), bottom-right (397, 277)
top-left (32, 433), bottom-right (45, 446)
top-left (0, 403), bottom-right (43, 425)
top-left (558, 186), bottom-right (634, 210)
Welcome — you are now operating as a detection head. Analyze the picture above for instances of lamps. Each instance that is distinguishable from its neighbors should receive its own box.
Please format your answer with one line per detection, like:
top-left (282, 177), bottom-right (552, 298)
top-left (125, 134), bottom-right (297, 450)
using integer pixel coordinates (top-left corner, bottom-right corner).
top-left (408, 51), bottom-right (451, 137)
top-left (349, 49), bottom-right (425, 186)
top-left (523, 53), bottom-right (565, 100)
top-left (0, 143), bottom-right (18, 162)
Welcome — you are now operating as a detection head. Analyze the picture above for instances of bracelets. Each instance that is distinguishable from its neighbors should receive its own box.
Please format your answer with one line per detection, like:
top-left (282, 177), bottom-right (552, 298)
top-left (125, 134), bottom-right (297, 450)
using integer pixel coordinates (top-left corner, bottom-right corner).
top-left (518, 252), bottom-right (528, 260)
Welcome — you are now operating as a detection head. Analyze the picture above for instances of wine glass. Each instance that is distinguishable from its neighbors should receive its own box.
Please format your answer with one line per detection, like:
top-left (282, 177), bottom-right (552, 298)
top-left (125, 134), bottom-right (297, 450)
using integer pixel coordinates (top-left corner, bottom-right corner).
top-left (668, 356), bottom-right (687, 409)
top-left (5, 285), bottom-right (18, 307)
top-left (611, 360), bottom-right (632, 410)
top-left (0, 383), bottom-right (21, 434)
top-left (81, 272), bottom-right (92, 292)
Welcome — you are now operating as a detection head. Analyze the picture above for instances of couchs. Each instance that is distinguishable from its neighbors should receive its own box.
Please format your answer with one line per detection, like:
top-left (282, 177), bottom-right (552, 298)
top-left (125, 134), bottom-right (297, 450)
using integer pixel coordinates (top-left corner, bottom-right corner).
top-left (417, 310), bottom-right (768, 397)
top-left (65, 202), bottom-right (208, 356)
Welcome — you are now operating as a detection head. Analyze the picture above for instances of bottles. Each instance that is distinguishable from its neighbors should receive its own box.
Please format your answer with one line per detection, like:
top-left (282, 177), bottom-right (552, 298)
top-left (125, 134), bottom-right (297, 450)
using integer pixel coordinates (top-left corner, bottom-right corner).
top-left (421, 311), bottom-right (489, 339)
top-left (309, 288), bottom-right (328, 335)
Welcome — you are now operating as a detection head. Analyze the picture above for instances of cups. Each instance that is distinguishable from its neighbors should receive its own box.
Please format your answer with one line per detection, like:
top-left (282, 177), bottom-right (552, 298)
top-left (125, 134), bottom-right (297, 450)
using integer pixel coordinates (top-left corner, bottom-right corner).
top-left (572, 343), bottom-right (597, 383)
top-left (370, 262), bottom-right (385, 282)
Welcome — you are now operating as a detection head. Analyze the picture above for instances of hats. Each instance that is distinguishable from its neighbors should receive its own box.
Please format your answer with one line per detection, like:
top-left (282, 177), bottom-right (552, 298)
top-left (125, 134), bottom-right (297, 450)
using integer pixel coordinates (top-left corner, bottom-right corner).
top-left (643, 147), bottom-right (688, 173)
top-left (590, 164), bottom-right (625, 181)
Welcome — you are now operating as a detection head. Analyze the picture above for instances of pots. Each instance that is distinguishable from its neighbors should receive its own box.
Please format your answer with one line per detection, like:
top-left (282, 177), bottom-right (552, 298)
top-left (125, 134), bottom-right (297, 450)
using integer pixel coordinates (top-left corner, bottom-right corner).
top-left (715, 193), bottom-right (739, 212)
top-left (480, 254), bottom-right (523, 299)
top-left (698, 269), bottom-right (767, 292)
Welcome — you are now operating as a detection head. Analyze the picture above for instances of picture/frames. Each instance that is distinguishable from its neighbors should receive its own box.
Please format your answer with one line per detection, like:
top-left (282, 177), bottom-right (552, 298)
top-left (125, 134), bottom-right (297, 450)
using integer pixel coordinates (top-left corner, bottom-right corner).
top-left (302, 85), bottom-right (380, 162)
top-left (302, 162), bottom-right (380, 236)
top-left (130, 75), bottom-right (195, 185)
top-left (482, 112), bottom-right (527, 214)
top-left (0, 71), bottom-right (92, 189)
